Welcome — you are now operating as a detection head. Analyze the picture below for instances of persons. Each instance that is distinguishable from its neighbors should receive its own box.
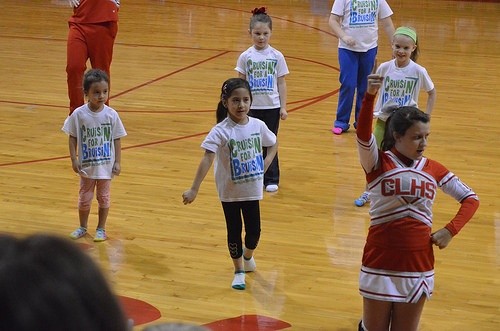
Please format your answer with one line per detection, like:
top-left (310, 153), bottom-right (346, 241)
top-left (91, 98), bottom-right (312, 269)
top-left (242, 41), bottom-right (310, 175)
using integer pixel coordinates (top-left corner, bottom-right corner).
top-left (0, 230), bottom-right (133, 331)
top-left (356, 56), bottom-right (481, 331)
top-left (180, 78), bottom-right (280, 291)
top-left (66, 0), bottom-right (120, 117)
top-left (329, 0), bottom-right (397, 135)
top-left (353, 25), bottom-right (436, 207)
top-left (234, 7), bottom-right (290, 193)
top-left (60, 68), bottom-right (127, 243)
